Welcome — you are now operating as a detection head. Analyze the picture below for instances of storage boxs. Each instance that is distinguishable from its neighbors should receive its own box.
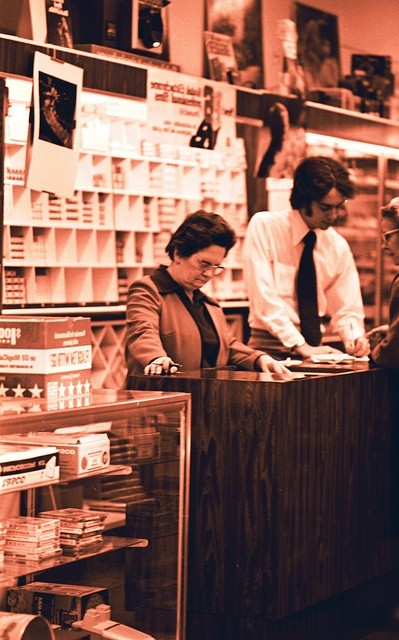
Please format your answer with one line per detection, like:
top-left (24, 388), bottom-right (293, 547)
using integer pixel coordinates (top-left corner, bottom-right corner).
top-left (1, 316), bottom-right (93, 401)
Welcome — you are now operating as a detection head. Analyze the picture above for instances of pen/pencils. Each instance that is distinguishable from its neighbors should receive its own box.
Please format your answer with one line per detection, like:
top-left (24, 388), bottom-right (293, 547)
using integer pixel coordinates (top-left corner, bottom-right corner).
top-left (152, 363), bottom-right (183, 368)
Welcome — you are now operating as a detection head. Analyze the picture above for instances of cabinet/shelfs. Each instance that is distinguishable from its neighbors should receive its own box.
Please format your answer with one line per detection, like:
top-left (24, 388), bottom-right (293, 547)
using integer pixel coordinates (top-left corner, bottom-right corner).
top-left (0, 387), bottom-right (191, 640)
top-left (240, 86), bottom-right (399, 345)
top-left (0, 34), bottom-right (250, 317)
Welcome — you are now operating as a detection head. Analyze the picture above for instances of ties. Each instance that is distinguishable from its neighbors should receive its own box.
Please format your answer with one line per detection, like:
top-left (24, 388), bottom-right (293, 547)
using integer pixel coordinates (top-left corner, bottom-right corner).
top-left (296, 231), bottom-right (322, 347)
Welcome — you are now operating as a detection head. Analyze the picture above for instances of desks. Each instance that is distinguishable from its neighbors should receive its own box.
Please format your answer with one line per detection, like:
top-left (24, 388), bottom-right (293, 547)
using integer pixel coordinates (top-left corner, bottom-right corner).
top-left (124, 349), bottom-right (399, 640)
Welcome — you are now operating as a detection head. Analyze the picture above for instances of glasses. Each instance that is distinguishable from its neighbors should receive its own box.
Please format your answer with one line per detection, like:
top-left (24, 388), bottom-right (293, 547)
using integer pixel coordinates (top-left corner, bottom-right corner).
top-left (382, 229), bottom-right (398, 240)
top-left (196, 254), bottom-right (225, 276)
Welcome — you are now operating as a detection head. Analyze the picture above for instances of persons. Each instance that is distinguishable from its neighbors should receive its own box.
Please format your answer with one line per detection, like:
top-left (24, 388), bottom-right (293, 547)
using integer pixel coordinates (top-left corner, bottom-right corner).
top-left (366, 199), bottom-right (399, 461)
top-left (126, 214), bottom-right (291, 380)
top-left (244, 158), bottom-right (372, 361)
